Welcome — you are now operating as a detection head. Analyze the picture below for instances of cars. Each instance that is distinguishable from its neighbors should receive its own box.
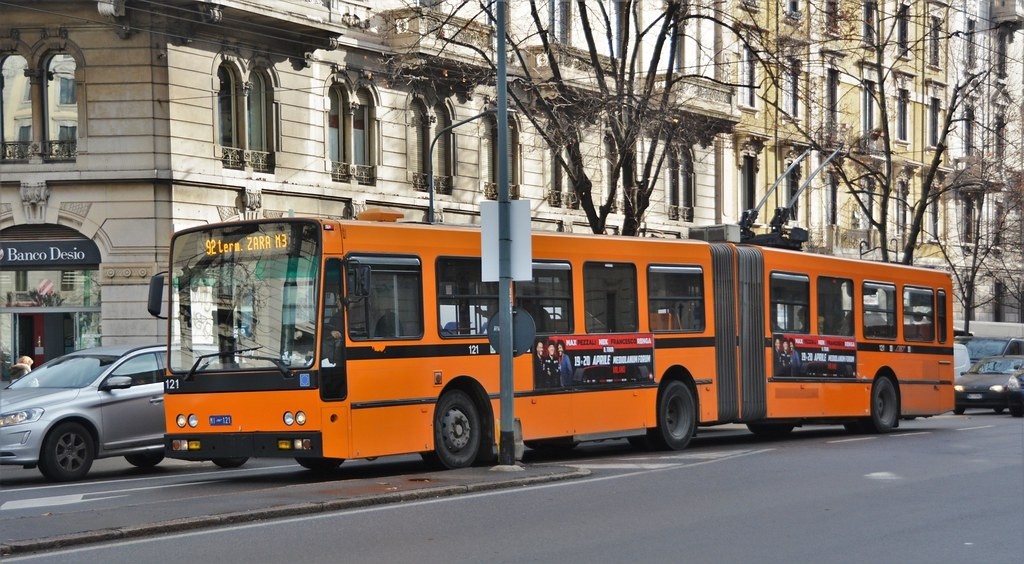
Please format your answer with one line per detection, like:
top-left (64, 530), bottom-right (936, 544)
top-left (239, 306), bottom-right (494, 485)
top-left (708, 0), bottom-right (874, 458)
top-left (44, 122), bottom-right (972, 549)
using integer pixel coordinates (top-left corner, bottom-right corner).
top-left (954, 354), bottom-right (1024, 418)
top-left (0, 344), bottom-right (250, 483)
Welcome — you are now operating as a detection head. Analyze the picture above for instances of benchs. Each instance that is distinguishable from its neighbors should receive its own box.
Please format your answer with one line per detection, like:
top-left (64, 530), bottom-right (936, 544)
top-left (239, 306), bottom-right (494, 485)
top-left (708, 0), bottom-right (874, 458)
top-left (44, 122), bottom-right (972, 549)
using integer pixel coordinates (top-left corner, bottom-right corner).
top-left (866, 323), bottom-right (933, 339)
top-left (651, 311), bottom-right (682, 330)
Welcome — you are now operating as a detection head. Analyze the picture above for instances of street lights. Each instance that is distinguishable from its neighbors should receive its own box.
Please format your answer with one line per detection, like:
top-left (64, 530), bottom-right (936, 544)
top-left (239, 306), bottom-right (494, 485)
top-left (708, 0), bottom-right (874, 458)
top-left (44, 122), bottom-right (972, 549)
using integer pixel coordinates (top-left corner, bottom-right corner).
top-left (427, 107), bottom-right (518, 222)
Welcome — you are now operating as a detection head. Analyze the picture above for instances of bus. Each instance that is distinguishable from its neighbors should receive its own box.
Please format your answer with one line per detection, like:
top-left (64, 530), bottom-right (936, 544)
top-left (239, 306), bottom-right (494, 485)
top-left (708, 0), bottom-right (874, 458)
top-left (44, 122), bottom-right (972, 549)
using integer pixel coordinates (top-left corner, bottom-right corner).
top-left (147, 143), bottom-right (956, 471)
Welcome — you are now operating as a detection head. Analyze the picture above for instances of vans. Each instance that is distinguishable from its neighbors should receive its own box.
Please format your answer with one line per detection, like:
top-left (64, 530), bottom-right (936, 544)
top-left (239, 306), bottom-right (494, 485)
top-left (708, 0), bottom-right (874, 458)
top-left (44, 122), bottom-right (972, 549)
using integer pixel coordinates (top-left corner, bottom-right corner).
top-left (953, 336), bottom-right (1024, 381)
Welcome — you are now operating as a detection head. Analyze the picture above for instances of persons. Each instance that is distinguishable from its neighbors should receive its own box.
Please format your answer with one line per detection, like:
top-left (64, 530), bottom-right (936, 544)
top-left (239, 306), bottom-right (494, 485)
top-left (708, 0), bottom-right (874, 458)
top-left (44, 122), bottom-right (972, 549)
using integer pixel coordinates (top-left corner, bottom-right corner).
top-left (534, 338), bottom-right (573, 388)
top-left (320, 289), bottom-right (386, 364)
top-left (775, 338), bottom-right (799, 377)
top-left (8, 356), bottom-right (33, 385)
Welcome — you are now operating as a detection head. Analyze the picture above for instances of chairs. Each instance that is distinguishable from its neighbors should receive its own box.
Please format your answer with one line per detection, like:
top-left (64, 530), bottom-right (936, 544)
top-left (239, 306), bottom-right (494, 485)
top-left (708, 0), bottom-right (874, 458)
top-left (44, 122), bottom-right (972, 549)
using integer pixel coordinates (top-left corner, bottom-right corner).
top-left (374, 312), bottom-right (404, 337)
